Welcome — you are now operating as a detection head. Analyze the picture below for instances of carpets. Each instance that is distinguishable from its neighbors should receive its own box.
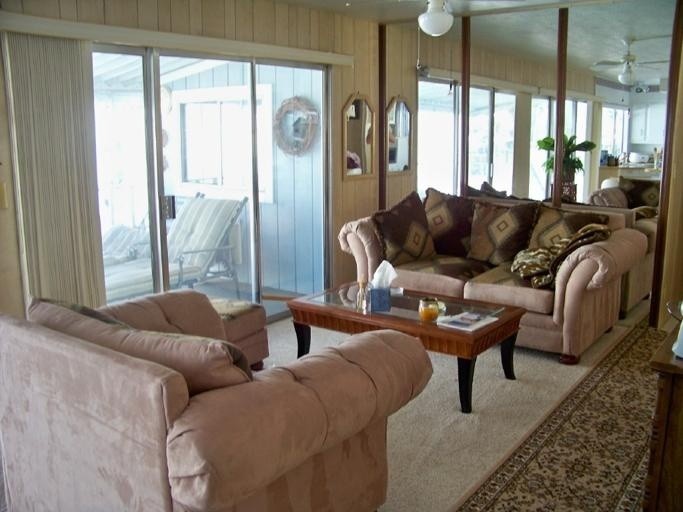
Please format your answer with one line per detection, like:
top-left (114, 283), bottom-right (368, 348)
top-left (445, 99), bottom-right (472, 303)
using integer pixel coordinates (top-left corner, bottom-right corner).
top-left (446, 313), bottom-right (681, 511)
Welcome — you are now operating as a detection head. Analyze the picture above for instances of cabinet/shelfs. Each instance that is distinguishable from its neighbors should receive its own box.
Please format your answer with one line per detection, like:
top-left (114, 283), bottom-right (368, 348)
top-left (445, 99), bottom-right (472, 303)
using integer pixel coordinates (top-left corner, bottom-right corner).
top-left (599, 102), bottom-right (667, 184)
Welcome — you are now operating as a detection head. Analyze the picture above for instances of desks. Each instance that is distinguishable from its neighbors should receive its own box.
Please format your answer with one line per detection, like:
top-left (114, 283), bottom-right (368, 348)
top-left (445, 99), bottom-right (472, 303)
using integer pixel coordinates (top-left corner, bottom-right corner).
top-left (640, 320), bottom-right (682, 512)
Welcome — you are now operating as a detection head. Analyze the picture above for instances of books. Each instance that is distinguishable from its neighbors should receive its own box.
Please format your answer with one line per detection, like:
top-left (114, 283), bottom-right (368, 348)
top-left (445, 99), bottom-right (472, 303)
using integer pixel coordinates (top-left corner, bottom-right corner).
top-left (436, 312), bottom-right (498, 330)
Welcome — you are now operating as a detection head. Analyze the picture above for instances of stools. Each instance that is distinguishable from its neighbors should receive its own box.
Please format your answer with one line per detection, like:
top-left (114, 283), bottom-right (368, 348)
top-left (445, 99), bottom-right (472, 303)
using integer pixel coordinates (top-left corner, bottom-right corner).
top-left (205, 294), bottom-right (271, 371)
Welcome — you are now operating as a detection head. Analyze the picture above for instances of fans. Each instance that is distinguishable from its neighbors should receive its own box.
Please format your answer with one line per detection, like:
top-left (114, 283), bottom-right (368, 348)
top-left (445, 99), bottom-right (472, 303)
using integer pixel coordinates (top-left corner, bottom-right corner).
top-left (587, 36), bottom-right (669, 73)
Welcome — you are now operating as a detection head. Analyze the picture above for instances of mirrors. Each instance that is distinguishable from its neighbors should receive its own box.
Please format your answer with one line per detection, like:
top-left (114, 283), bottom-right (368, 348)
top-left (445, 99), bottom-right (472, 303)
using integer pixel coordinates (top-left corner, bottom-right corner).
top-left (340, 90), bottom-right (376, 181)
top-left (462, 7), bottom-right (557, 208)
top-left (376, 10), bottom-right (461, 208)
top-left (558, 0), bottom-right (683, 335)
top-left (383, 92), bottom-right (415, 176)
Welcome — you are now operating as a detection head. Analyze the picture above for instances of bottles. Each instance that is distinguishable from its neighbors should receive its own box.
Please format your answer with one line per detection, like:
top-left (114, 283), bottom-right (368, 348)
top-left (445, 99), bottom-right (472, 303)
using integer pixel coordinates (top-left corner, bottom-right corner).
top-left (356, 282), bottom-right (367, 310)
top-left (622, 152), bottom-right (626, 163)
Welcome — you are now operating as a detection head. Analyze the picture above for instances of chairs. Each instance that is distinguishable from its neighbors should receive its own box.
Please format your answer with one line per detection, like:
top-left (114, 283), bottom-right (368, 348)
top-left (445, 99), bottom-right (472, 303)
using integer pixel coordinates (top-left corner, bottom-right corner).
top-left (102, 186), bottom-right (253, 307)
top-left (0, 281), bottom-right (436, 511)
top-left (101, 223), bottom-right (139, 268)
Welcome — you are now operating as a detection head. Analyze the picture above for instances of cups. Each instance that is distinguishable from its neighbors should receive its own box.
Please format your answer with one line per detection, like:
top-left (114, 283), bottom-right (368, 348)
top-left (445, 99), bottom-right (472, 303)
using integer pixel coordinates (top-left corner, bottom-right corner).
top-left (418, 297), bottom-right (438, 322)
top-left (607, 156), bottom-right (615, 165)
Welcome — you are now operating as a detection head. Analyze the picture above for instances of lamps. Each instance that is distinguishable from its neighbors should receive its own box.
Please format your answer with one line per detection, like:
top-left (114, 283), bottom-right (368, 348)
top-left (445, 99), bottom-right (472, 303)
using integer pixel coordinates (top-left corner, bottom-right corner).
top-left (414, 2), bottom-right (458, 39)
top-left (612, 68), bottom-right (640, 87)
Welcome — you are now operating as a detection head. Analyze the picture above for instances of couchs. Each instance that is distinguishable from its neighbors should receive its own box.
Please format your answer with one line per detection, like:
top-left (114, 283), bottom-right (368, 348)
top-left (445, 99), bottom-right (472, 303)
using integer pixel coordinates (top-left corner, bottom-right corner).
top-left (336, 190), bottom-right (650, 368)
top-left (537, 195), bottom-right (659, 321)
top-left (590, 177), bottom-right (662, 215)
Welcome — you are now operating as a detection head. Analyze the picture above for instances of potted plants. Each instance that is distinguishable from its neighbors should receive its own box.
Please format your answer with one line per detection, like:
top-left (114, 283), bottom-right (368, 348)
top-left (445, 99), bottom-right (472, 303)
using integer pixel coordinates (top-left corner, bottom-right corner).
top-left (536, 133), bottom-right (598, 184)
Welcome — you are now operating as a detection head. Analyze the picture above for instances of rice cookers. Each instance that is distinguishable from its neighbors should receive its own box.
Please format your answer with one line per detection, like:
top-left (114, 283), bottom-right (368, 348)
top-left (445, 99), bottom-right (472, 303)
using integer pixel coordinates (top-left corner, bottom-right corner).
top-left (629, 152), bottom-right (649, 163)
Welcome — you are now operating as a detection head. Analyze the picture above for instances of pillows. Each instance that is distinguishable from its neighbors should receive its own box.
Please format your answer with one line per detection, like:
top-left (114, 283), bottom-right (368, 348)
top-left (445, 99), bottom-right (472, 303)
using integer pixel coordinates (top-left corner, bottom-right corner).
top-left (424, 186), bottom-right (478, 260)
top-left (369, 192), bottom-right (439, 266)
top-left (630, 203), bottom-right (658, 221)
top-left (458, 181), bottom-right (536, 201)
top-left (523, 204), bottom-right (611, 252)
top-left (21, 291), bottom-right (266, 386)
top-left (617, 176), bottom-right (661, 207)
top-left (465, 200), bottom-right (542, 268)
